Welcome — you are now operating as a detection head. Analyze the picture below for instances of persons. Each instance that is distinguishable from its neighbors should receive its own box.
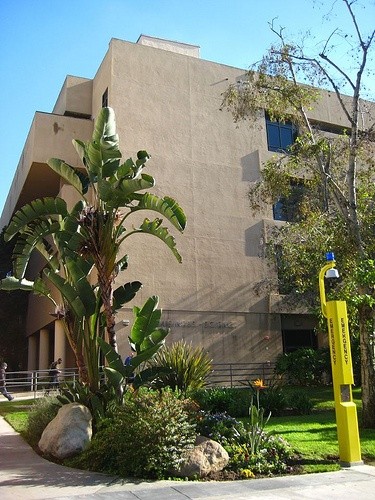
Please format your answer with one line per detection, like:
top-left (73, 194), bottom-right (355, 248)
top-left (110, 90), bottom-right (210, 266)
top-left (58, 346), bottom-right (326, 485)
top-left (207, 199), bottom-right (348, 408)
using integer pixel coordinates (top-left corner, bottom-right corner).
top-left (44, 357), bottom-right (63, 395)
top-left (0, 363), bottom-right (15, 402)
top-left (124, 353), bottom-right (134, 367)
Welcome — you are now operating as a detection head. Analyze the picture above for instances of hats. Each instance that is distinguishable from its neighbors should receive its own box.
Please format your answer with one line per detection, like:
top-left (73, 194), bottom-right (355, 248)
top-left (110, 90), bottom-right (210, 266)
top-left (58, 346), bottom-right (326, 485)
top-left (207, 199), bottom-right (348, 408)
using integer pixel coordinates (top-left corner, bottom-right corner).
top-left (1, 362), bottom-right (8, 366)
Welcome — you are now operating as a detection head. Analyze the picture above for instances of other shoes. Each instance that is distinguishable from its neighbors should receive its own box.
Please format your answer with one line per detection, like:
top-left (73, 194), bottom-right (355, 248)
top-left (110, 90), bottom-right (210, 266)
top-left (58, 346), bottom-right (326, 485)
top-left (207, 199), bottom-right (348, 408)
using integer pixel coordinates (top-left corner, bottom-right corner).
top-left (9, 396), bottom-right (16, 401)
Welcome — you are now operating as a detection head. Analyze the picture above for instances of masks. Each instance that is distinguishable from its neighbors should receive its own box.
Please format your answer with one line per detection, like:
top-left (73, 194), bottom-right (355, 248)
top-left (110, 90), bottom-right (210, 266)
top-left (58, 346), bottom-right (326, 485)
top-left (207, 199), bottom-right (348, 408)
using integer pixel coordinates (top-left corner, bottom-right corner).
top-left (4, 366), bottom-right (7, 369)
top-left (59, 362), bottom-right (61, 364)
